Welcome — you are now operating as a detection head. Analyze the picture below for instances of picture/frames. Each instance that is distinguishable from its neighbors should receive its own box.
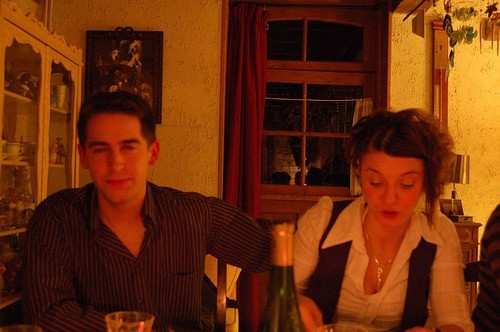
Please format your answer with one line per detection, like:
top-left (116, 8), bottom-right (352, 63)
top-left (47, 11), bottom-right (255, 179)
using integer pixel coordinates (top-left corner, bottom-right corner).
top-left (84, 26), bottom-right (166, 123)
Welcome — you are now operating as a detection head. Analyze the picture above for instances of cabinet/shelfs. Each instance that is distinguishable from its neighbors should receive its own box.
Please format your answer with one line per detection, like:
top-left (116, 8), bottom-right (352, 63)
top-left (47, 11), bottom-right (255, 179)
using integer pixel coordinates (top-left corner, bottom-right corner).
top-left (453, 219), bottom-right (483, 304)
top-left (0, 0), bottom-right (86, 309)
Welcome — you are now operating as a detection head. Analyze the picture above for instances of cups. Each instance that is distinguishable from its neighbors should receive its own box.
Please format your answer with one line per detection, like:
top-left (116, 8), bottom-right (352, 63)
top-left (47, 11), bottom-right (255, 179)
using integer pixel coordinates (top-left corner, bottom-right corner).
top-left (6, 142), bottom-right (24, 161)
top-left (105, 311), bottom-right (155, 332)
top-left (0, 324), bottom-right (42, 332)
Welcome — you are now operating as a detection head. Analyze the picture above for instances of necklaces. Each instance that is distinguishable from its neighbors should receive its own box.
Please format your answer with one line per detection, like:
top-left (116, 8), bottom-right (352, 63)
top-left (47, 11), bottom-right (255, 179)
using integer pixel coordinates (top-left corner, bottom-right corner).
top-left (362, 217), bottom-right (399, 290)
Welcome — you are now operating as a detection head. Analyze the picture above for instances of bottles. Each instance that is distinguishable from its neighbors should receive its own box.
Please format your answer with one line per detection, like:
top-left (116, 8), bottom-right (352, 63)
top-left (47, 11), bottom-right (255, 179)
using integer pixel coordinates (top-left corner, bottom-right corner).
top-left (256, 218), bottom-right (307, 332)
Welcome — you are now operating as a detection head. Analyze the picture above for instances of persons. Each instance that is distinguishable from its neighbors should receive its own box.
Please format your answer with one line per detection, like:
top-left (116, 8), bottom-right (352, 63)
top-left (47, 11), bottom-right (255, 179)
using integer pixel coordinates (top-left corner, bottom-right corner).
top-left (471, 203), bottom-right (500, 331)
top-left (16, 89), bottom-right (295, 332)
top-left (280, 108), bottom-right (476, 332)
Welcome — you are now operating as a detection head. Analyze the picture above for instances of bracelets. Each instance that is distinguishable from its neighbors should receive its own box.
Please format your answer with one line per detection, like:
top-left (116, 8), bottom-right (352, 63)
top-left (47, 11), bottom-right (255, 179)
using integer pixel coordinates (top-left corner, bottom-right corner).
top-left (432, 326), bottom-right (442, 332)
